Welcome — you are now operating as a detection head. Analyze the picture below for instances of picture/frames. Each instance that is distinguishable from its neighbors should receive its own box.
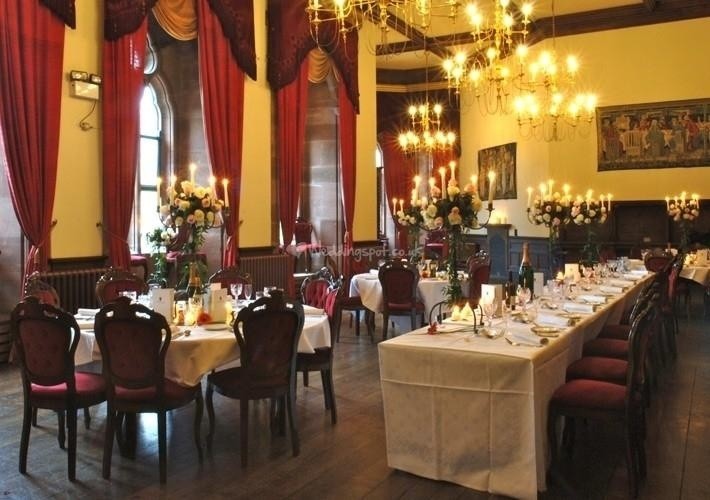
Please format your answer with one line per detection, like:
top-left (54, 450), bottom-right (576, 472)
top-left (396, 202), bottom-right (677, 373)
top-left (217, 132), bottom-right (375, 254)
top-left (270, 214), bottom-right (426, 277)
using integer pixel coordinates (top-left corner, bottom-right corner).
top-left (595, 98), bottom-right (710, 172)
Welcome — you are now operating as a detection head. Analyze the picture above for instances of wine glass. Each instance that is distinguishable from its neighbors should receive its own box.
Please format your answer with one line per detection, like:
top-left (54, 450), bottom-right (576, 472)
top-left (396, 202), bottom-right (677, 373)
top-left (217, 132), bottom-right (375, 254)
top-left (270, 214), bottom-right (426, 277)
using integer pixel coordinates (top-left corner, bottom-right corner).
top-left (230, 284), bottom-right (283, 308)
top-left (481, 255), bottom-right (628, 333)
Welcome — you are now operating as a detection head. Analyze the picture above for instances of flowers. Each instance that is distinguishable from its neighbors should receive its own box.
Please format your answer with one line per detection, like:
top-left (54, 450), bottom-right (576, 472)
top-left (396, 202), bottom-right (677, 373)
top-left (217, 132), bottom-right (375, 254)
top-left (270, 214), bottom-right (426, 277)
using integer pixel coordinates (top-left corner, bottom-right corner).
top-left (158, 182), bottom-right (225, 228)
top-left (571, 199), bottom-right (607, 227)
top-left (530, 192), bottom-right (573, 228)
top-left (668, 200), bottom-right (699, 221)
top-left (397, 183), bottom-right (484, 233)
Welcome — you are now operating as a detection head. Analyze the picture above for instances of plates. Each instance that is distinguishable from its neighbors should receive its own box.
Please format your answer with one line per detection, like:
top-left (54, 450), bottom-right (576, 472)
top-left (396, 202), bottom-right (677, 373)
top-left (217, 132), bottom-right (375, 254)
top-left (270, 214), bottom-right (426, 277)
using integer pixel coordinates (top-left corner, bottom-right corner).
top-left (531, 326), bottom-right (562, 338)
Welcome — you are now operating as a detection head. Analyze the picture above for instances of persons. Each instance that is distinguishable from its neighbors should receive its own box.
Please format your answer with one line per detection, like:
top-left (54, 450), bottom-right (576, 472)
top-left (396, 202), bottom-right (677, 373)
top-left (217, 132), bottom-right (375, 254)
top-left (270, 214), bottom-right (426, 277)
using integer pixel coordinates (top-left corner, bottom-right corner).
top-left (603, 110), bottom-right (709, 168)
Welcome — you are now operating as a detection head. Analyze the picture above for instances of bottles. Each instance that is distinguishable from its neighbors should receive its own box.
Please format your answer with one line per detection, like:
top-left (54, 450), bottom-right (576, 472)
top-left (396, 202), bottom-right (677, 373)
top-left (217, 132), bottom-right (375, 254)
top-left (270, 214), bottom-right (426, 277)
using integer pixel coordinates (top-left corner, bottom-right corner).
top-left (421, 263), bottom-right (437, 278)
top-left (518, 241), bottom-right (534, 301)
top-left (174, 261), bottom-right (201, 326)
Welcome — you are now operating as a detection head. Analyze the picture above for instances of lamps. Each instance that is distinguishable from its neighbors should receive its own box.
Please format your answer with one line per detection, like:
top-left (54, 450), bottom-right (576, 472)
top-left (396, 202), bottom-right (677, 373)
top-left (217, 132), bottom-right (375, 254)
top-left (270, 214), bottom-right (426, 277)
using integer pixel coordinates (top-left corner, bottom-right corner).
top-left (70, 70), bottom-right (103, 131)
